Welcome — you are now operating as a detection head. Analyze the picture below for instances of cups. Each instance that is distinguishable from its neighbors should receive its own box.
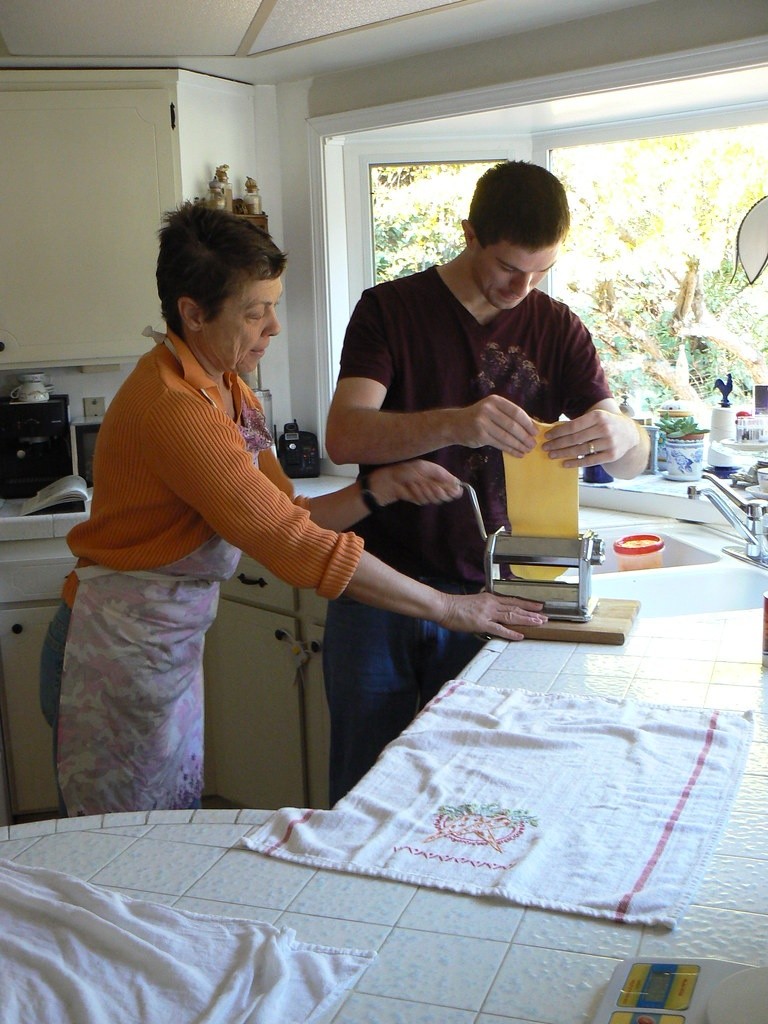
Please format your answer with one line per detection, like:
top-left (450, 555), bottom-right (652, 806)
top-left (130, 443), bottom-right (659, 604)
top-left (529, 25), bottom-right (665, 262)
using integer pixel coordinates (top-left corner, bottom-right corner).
top-left (10, 382), bottom-right (54, 402)
top-left (614, 534), bottom-right (665, 571)
top-left (666, 438), bottom-right (704, 475)
top-left (757, 468), bottom-right (768, 492)
top-left (18, 373), bottom-right (46, 382)
top-left (736, 415), bottom-right (768, 442)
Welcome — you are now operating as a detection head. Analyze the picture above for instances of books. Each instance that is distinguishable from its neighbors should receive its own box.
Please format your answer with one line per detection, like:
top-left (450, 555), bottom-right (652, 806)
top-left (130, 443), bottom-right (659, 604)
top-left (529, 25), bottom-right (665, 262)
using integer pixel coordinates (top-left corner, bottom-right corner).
top-left (20, 475), bottom-right (93, 516)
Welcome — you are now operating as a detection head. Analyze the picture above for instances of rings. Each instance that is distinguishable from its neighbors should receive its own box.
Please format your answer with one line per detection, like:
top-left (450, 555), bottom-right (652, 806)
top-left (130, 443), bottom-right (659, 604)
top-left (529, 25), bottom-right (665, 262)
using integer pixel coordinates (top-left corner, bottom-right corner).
top-left (587, 442), bottom-right (597, 455)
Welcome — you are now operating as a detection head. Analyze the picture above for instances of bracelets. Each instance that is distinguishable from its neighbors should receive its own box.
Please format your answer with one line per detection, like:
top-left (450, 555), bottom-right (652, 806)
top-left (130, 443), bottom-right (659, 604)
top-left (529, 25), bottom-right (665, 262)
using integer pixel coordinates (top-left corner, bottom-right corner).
top-left (357, 471), bottom-right (385, 516)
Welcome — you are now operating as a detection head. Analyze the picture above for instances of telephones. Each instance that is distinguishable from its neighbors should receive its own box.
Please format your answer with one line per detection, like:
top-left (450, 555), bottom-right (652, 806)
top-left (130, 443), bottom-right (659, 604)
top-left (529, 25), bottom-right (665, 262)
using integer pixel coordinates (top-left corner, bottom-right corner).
top-left (278, 418), bottom-right (321, 479)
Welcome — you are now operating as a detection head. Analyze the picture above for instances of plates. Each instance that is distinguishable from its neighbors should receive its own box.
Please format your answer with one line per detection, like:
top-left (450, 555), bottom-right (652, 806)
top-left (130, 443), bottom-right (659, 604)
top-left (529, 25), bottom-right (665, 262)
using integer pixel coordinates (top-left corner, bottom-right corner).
top-left (661, 471), bottom-right (702, 481)
top-left (745, 485), bottom-right (768, 499)
top-left (721, 438), bottom-right (768, 451)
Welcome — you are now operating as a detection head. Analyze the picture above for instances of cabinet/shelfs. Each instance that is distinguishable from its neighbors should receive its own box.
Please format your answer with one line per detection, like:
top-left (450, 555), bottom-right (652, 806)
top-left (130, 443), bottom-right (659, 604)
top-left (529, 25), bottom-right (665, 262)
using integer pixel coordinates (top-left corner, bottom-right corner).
top-left (0, 605), bottom-right (63, 816)
top-left (0, 70), bottom-right (254, 370)
top-left (202, 556), bottom-right (332, 810)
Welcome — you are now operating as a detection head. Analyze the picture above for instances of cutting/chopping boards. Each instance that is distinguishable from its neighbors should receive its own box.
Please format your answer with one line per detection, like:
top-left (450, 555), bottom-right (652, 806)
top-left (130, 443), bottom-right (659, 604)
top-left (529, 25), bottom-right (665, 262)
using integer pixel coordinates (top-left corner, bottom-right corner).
top-left (498, 600), bottom-right (640, 645)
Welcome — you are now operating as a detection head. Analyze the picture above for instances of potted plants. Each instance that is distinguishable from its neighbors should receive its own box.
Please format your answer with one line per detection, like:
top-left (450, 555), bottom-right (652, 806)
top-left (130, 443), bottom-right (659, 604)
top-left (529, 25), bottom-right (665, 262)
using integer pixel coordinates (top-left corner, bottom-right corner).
top-left (653, 412), bottom-right (710, 481)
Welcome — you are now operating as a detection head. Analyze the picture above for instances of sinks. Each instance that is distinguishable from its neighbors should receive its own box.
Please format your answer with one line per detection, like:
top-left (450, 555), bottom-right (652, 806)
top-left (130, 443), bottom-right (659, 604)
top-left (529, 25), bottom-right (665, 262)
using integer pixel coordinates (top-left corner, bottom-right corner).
top-left (554, 521), bottom-right (727, 580)
top-left (552, 564), bottom-right (768, 621)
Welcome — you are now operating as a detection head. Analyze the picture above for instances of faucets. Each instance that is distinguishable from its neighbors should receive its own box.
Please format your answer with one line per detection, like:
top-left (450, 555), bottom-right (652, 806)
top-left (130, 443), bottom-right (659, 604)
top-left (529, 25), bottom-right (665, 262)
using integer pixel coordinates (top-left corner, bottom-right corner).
top-left (686, 485), bottom-right (768, 560)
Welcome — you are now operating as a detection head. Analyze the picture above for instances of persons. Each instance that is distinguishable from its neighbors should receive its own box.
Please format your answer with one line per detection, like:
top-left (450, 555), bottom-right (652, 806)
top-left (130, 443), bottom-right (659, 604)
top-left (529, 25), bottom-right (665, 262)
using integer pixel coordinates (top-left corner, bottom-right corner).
top-left (323, 159), bottom-right (651, 811)
top-left (38, 199), bottom-right (548, 818)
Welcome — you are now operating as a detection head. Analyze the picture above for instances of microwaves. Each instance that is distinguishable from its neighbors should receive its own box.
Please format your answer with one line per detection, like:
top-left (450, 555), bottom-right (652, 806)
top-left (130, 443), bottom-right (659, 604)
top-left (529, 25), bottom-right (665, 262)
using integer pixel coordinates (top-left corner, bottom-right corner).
top-left (70, 416), bottom-right (104, 487)
